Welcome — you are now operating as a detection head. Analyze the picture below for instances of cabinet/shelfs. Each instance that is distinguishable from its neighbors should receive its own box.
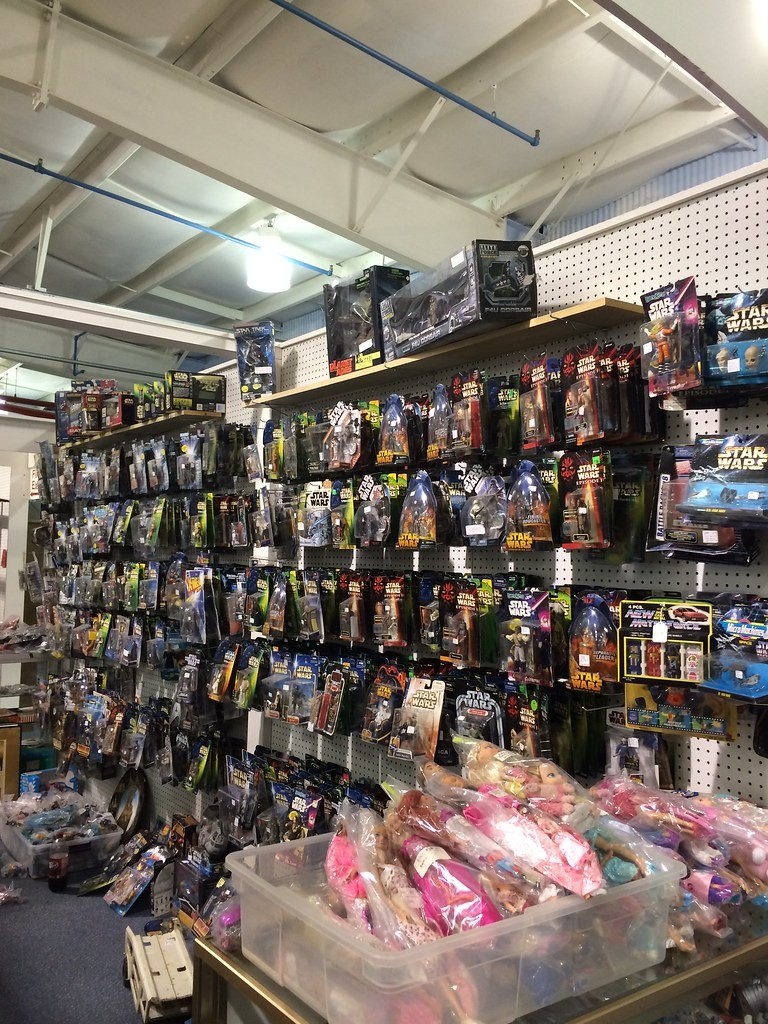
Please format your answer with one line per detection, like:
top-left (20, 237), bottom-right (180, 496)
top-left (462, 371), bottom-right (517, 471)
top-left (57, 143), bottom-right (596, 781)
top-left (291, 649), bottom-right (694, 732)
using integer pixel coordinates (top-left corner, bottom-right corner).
top-left (191, 904), bottom-right (767, 1024)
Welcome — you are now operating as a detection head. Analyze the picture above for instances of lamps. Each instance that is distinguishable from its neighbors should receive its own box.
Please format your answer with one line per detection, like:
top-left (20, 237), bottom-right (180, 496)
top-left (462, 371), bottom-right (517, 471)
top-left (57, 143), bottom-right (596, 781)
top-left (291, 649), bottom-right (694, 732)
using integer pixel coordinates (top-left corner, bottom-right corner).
top-left (246, 213), bottom-right (290, 293)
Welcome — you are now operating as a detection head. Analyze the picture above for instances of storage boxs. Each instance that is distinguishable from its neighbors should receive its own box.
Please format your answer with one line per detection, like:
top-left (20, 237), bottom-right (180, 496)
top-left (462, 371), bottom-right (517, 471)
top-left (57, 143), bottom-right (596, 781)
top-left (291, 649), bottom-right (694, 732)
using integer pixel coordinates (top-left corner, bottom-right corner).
top-left (55, 369), bottom-right (226, 446)
top-left (169, 814), bottom-right (226, 919)
top-left (0, 800), bottom-right (124, 878)
top-left (616, 288), bottom-right (768, 702)
top-left (224, 832), bottom-right (688, 1023)
top-left (323, 240), bottom-right (538, 378)
top-left (234, 320), bottom-right (283, 401)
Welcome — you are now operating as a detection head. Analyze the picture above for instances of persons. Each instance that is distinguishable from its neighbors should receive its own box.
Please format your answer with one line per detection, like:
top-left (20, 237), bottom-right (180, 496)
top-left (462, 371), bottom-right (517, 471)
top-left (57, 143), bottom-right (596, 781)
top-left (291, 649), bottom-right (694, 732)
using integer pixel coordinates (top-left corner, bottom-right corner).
top-left (333, 739), bottom-right (766, 1024)
top-left (644, 318), bottom-right (678, 374)
top-left (483, 480), bottom-right (504, 501)
top-left (501, 626), bottom-right (531, 673)
top-left (470, 500), bottom-right (487, 525)
